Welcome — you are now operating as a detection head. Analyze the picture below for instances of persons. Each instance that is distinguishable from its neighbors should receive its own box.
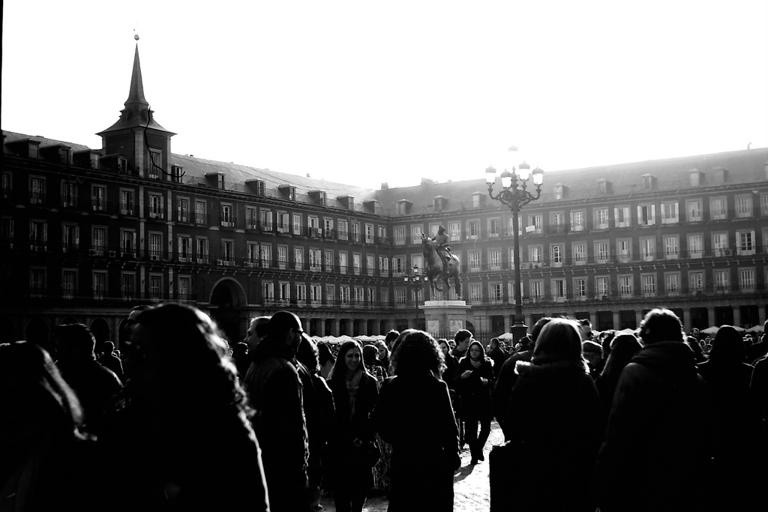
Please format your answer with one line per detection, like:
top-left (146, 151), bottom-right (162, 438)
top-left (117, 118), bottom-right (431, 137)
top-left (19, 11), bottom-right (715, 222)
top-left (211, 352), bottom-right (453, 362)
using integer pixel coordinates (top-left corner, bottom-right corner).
top-left (1, 301), bottom-right (767, 511)
top-left (432, 225), bottom-right (451, 275)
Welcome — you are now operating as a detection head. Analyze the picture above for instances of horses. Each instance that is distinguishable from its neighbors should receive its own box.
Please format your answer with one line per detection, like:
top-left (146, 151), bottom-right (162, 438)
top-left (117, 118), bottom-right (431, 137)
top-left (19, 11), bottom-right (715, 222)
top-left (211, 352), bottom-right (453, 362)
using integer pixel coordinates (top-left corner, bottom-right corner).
top-left (421, 237), bottom-right (462, 299)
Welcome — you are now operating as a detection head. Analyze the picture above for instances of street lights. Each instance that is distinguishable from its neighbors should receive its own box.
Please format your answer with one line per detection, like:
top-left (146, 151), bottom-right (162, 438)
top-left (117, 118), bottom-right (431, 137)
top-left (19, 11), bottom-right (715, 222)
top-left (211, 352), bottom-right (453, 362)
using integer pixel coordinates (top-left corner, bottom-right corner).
top-left (486, 161), bottom-right (543, 346)
top-left (402, 264), bottom-right (429, 329)
